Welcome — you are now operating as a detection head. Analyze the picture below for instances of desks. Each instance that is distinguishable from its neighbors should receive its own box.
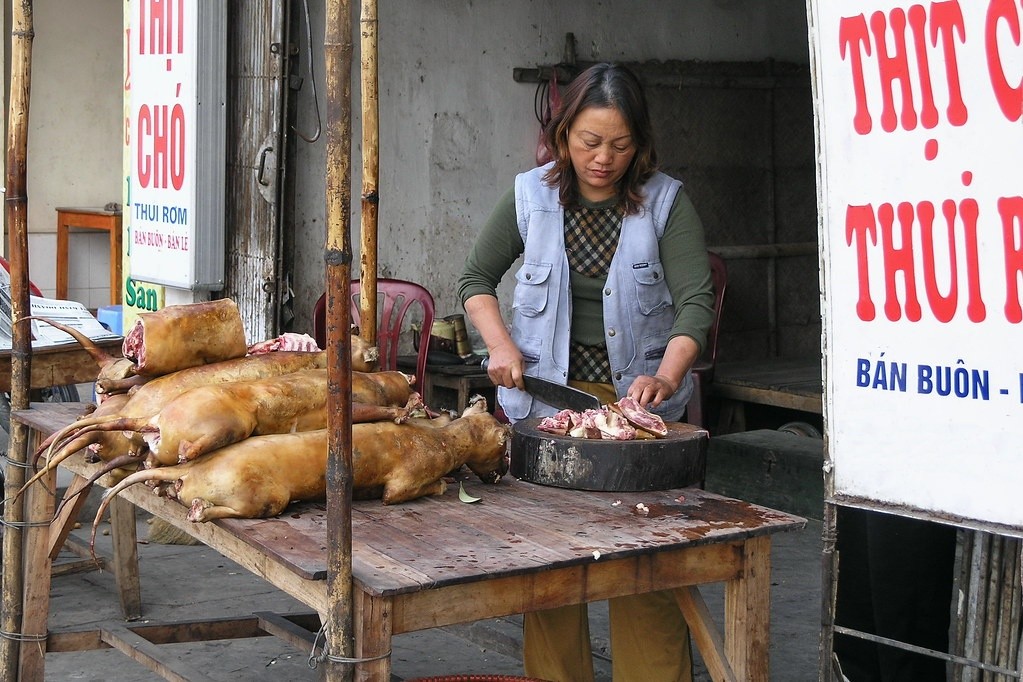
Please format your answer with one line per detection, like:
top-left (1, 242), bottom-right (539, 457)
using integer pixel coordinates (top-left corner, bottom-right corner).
top-left (0, 335), bottom-right (125, 535)
top-left (11, 402), bottom-right (810, 682)
top-left (55, 206), bottom-right (121, 323)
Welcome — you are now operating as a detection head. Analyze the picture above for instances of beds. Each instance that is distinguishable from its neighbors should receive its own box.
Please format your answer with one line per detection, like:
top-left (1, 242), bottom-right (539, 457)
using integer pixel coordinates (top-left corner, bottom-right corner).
top-left (706, 362), bottom-right (823, 439)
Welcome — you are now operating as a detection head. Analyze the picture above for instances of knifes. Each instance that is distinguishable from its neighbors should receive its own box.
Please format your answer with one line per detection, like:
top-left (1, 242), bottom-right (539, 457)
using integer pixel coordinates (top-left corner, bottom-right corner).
top-left (479, 358), bottom-right (601, 416)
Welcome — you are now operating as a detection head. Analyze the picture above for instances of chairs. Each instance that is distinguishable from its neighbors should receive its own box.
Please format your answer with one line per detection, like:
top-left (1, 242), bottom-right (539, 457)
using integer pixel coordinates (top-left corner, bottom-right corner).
top-left (313, 278), bottom-right (436, 403)
top-left (682, 250), bottom-right (729, 491)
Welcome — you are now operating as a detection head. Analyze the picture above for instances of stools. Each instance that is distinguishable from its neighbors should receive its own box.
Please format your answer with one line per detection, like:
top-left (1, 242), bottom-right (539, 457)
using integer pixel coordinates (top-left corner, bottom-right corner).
top-left (92, 305), bottom-right (124, 405)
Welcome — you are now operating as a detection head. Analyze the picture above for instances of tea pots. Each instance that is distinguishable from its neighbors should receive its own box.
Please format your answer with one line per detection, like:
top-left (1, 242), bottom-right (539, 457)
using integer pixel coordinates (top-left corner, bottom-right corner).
top-left (409, 318), bottom-right (455, 355)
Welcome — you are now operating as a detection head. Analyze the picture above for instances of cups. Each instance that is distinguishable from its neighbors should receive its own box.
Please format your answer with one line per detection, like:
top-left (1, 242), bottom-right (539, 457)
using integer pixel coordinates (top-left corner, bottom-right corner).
top-left (446, 315), bottom-right (468, 355)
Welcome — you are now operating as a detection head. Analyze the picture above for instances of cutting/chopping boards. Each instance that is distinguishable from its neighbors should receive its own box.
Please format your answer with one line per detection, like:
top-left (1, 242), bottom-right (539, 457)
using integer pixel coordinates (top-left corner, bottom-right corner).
top-left (512, 414), bottom-right (705, 489)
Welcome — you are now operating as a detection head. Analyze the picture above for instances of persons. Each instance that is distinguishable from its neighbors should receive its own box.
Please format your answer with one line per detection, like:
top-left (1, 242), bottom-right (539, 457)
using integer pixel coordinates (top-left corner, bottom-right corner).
top-left (454, 62), bottom-right (718, 681)
